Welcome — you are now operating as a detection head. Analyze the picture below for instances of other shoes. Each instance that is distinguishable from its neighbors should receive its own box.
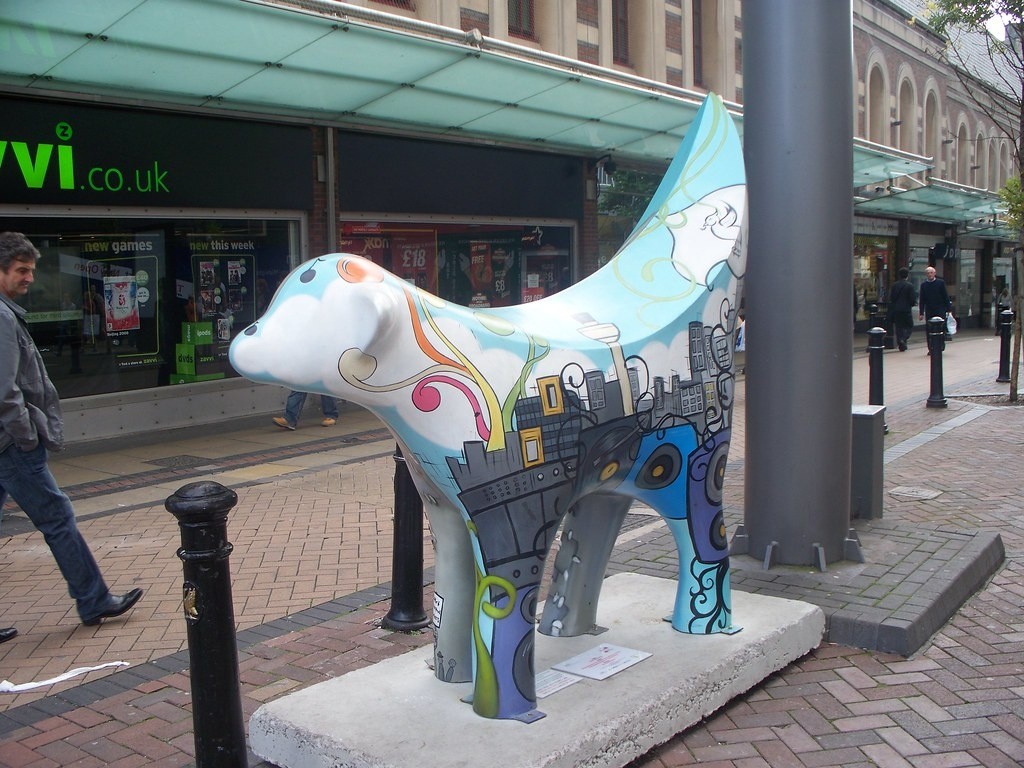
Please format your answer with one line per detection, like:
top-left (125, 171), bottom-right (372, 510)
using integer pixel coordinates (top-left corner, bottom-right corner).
top-left (898, 340), bottom-right (907, 351)
top-left (272, 418), bottom-right (295, 431)
top-left (320, 418), bottom-right (336, 427)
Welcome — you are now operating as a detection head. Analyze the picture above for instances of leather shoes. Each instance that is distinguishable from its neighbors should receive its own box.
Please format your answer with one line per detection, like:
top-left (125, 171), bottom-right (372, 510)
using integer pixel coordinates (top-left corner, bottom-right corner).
top-left (83, 588), bottom-right (143, 626)
top-left (0, 628), bottom-right (17, 643)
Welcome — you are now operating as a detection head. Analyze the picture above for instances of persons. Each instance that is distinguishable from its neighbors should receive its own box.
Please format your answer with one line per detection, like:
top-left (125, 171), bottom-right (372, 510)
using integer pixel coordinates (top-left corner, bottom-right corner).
top-left (0, 232), bottom-right (143, 642)
top-left (271, 391), bottom-right (338, 431)
top-left (993, 287), bottom-right (1014, 335)
top-left (919, 267), bottom-right (951, 355)
top-left (890, 267), bottom-right (916, 351)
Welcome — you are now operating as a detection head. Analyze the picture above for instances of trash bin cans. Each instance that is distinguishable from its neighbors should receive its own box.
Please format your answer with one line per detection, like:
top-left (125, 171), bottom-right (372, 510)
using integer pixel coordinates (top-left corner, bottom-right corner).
top-left (996, 305), bottom-right (1010, 336)
top-left (853, 404), bottom-right (888, 520)
top-left (870, 302), bottom-right (898, 349)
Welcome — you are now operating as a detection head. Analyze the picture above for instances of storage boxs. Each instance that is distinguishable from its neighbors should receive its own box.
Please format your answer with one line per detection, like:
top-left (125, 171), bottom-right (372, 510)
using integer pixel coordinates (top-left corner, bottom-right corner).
top-left (168, 323), bottom-right (225, 384)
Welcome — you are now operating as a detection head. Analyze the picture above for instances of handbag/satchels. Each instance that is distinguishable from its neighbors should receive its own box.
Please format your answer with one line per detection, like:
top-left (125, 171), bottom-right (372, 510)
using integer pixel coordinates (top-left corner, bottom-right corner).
top-left (887, 305), bottom-right (895, 323)
top-left (946, 315), bottom-right (957, 335)
